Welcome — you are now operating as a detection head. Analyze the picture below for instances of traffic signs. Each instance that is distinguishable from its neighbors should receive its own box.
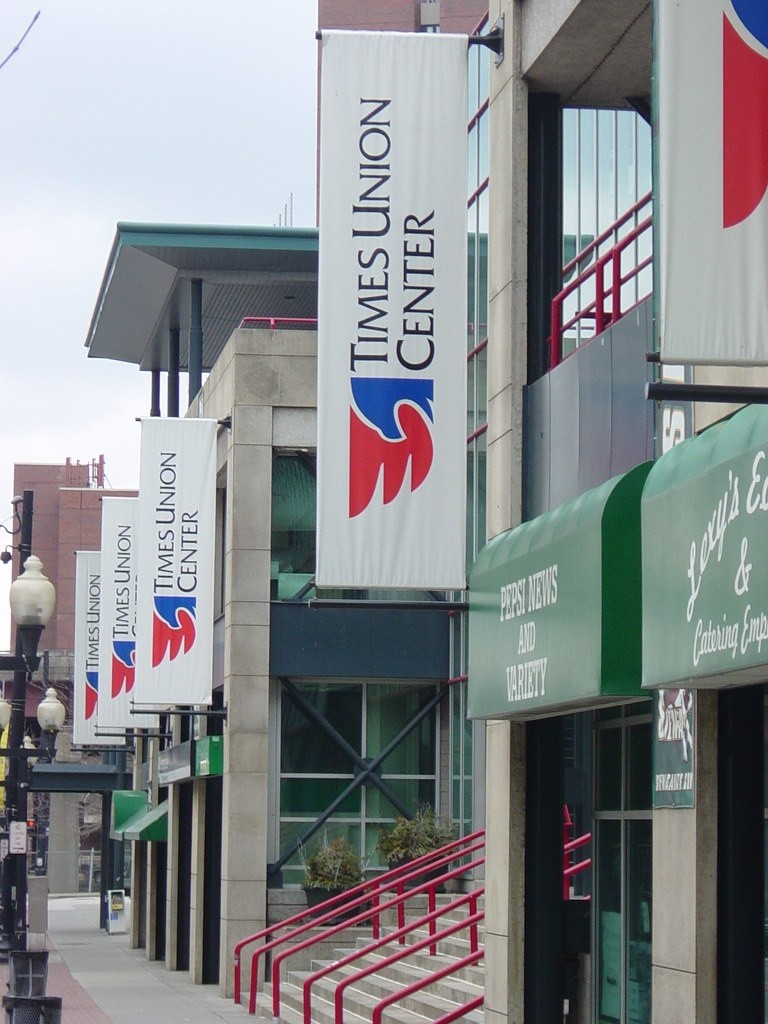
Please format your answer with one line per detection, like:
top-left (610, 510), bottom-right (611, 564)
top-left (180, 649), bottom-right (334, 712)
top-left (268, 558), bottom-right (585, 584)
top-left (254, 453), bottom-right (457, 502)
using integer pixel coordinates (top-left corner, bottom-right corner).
top-left (9, 820), bottom-right (27, 855)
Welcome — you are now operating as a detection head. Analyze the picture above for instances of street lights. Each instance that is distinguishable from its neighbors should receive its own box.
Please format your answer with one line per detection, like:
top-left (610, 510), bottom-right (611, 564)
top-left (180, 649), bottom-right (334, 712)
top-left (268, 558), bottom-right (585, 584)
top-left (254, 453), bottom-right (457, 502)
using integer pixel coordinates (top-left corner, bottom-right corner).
top-left (0, 554), bottom-right (67, 961)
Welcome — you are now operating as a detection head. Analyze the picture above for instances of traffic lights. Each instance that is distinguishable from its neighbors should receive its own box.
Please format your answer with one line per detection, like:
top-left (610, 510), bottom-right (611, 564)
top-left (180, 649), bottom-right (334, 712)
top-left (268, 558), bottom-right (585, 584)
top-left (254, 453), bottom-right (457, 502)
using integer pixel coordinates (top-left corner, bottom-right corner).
top-left (27, 819), bottom-right (37, 829)
